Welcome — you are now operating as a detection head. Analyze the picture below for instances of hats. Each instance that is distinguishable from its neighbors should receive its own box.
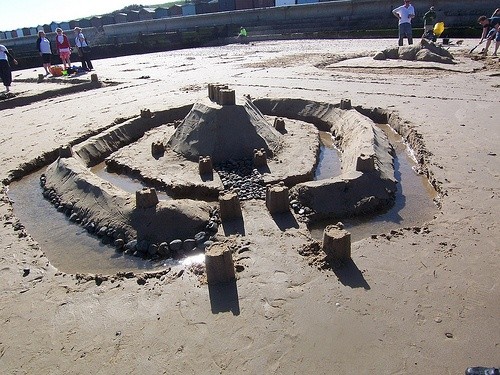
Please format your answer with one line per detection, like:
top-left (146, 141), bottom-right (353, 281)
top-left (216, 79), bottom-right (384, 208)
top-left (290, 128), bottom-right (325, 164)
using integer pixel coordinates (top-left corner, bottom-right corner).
top-left (240, 26), bottom-right (244, 29)
top-left (404, 0), bottom-right (412, 2)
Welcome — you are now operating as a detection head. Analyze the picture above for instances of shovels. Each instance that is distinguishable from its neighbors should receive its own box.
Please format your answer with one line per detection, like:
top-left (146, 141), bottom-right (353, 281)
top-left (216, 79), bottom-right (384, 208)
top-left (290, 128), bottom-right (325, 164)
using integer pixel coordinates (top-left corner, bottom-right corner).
top-left (470, 38), bottom-right (489, 53)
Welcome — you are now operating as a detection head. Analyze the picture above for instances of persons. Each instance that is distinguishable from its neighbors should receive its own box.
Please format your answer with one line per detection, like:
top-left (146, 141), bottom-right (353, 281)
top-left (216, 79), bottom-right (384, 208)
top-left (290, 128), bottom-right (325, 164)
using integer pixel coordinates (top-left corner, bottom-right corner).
top-left (55, 27), bottom-right (72, 69)
top-left (237, 26), bottom-right (247, 38)
top-left (0, 45), bottom-right (18, 92)
top-left (479, 16), bottom-right (500, 56)
top-left (422, 6), bottom-right (435, 38)
top-left (36, 31), bottom-right (53, 75)
top-left (73, 27), bottom-right (93, 70)
top-left (392, 0), bottom-right (415, 46)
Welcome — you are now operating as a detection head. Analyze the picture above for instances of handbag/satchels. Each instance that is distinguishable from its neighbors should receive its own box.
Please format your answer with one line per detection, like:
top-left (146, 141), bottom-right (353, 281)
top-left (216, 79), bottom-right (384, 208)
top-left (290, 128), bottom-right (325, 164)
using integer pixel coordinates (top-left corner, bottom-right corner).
top-left (82, 46), bottom-right (91, 54)
top-left (77, 49), bottom-right (83, 57)
